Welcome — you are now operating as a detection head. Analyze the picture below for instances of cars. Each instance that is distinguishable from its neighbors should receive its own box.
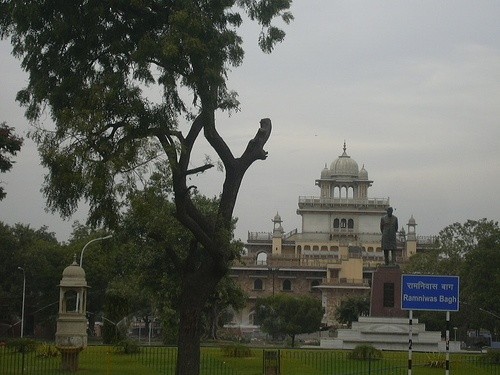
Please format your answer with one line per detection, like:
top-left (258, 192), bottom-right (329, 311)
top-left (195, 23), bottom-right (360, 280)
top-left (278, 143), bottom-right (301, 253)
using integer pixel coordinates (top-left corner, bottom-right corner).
top-left (465, 328), bottom-right (492, 347)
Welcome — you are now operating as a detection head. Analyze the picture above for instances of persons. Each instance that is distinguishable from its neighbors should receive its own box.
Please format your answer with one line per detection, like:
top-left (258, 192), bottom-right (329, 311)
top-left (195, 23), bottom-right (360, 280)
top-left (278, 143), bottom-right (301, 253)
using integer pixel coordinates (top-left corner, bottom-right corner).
top-left (380, 207), bottom-right (399, 264)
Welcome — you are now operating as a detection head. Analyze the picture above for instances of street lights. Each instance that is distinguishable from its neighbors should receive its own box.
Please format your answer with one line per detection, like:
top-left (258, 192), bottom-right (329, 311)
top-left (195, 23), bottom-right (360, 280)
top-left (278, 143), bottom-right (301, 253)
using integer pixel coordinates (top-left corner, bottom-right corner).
top-left (75, 235), bottom-right (114, 312)
top-left (17, 266), bottom-right (25, 337)
